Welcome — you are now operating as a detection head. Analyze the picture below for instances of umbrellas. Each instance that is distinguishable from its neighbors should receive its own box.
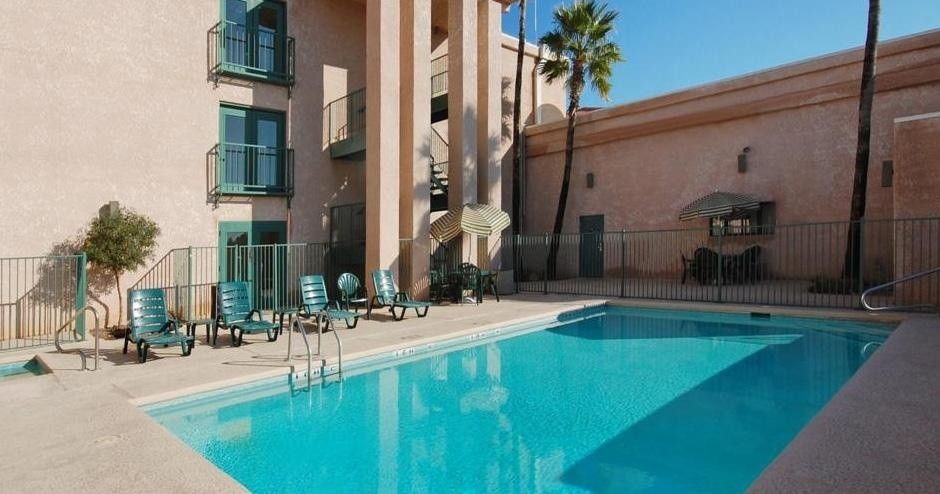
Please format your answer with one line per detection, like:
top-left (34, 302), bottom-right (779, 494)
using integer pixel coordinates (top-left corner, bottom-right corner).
top-left (429, 202), bottom-right (510, 243)
top-left (679, 192), bottom-right (759, 221)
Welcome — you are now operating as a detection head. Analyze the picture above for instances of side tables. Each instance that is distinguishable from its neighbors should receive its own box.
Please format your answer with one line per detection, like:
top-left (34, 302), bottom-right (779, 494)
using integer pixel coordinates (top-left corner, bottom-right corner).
top-left (274, 307), bottom-right (303, 334)
top-left (189, 319), bottom-right (216, 347)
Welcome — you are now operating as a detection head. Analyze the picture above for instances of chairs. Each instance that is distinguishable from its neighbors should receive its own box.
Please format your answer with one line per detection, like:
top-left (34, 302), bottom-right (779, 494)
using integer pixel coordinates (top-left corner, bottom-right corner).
top-left (429, 263), bottom-right (502, 305)
top-left (338, 273), bottom-right (370, 320)
top-left (368, 270), bottom-right (430, 320)
top-left (299, 275), bottom-right (360, 333)
top-left (213, 280), bottom-right (280, 347)
top-left (123, 287), bottom-right (194, 363)
top-left (679, 242), bottom-right (761, 285)
